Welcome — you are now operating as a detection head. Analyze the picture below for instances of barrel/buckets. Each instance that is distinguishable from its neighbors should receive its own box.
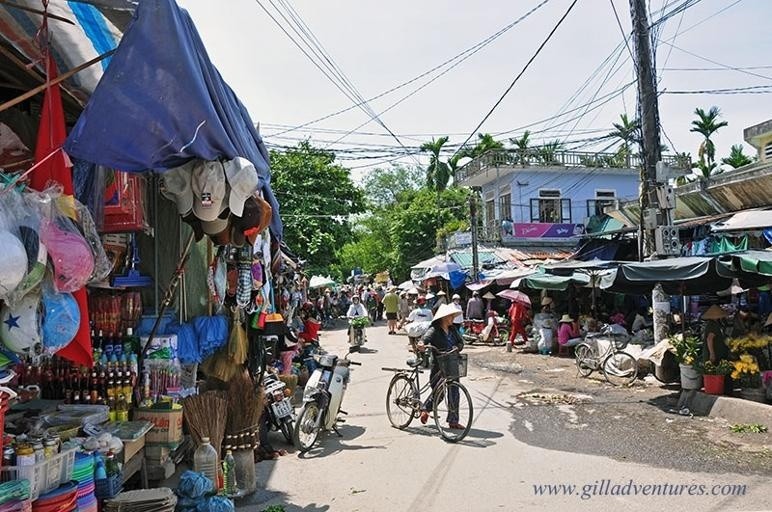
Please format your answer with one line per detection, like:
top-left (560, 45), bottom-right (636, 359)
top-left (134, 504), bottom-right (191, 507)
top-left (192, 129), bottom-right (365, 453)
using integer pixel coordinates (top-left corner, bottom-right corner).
top-left (193, 436), bottom-right (220, 492)
top-left (33, 455), bottom-right (100, 512)
top-left (677, 363), bottom-right (703, 391)
top-left (303, 358), bottom-right (317, 374)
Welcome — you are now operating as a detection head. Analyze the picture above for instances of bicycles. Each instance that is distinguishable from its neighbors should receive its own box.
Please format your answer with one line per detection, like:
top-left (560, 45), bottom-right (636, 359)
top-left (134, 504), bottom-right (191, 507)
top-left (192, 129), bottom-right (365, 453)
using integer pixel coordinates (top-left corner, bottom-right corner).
top-left (383, 343), bottom-right (475, 443)
top-left (575, 322), bottom-right (639, 388)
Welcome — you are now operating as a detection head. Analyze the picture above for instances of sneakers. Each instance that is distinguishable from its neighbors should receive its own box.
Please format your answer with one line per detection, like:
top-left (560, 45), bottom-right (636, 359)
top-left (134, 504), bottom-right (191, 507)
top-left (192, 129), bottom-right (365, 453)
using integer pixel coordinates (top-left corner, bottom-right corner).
top-left (421, 410), bottom-right (429, 424)
top-left (450, 424), bottom-right (465, 430)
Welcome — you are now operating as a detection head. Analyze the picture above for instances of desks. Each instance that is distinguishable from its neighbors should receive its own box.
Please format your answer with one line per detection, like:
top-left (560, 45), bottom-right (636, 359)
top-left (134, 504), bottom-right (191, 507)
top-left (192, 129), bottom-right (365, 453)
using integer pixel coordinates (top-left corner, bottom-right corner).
top-left (585, 333), bottom-right (654, 377)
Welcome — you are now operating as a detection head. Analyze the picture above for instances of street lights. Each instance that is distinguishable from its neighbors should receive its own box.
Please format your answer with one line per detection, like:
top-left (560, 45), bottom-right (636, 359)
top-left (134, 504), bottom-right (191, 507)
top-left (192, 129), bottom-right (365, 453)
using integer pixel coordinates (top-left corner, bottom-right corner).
top-left (468, 183), bottom-right (483, 277)
top-left (392, 161), bottom-right (444, 252)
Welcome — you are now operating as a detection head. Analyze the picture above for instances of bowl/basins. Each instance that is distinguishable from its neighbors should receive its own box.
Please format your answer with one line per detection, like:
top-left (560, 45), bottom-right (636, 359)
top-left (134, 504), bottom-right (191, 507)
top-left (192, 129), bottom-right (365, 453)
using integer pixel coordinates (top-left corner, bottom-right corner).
top-left (45, 423), bottom-right (82, 439)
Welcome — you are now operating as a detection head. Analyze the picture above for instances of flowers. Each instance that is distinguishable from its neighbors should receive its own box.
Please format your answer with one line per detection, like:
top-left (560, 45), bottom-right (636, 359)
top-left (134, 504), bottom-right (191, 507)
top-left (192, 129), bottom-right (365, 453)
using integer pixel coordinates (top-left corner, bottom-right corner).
top-left (668, 328), bottom-right (772, 405)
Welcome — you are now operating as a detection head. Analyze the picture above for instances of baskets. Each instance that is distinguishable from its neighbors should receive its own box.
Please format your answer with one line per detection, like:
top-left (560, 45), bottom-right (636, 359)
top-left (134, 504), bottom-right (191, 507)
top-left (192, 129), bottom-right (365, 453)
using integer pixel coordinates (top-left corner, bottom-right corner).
top-left (45, 424), bottom-right (83, 442)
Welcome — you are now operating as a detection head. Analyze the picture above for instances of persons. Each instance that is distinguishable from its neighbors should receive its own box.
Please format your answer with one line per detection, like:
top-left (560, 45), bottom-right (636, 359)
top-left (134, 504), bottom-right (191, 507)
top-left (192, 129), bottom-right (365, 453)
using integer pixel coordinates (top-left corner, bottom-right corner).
top-left (631, 305), bottom-right (652, 333)
top-left (314, 279), bottom-right (496, 430)
top-left (701, 303), bottom-right (727, 364)
top-left (507, 295), bottom-right (584, 356)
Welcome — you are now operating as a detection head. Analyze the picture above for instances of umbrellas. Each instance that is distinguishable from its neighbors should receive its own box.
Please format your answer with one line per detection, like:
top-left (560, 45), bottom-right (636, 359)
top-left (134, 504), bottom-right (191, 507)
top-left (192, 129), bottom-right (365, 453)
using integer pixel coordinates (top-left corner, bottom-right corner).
top-left (498, 289), bottom-right (533, 309)
top-left (540, 257), bottom-right (636, 317)
top-left (730, 249), bottom-right (772, 277)
top-left (604, 256), bottom-right (734, 344)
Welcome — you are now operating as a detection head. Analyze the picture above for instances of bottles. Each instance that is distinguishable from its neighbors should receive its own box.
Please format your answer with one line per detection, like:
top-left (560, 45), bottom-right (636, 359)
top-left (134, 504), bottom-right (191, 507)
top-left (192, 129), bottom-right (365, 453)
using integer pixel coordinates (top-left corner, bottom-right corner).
top-left (195, 434), bottom-right (219, 495)
top-left (222, 449), bottom-right (239, 495)
top-left (94, 446), bottom-right (118, 477)
top-left (20, 325), bottom-right (138, 422)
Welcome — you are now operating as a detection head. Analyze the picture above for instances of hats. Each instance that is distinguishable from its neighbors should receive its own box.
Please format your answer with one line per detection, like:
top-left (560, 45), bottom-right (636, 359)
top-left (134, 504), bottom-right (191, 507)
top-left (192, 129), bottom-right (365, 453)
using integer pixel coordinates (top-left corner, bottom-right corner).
top-left (542, 319), bottom-right (553, 328)
top-left (385, 285), bottom-right (461, 300)
top-left (610, 313), bottom-right (627, 325)
top-left (430, 303), bottom-right (462, 325)
top-left (0, 191), bottom-right (114, 360)
top-left (541, 297), bottom-right (553, 306)
top-left (157, 156), bottom-right (273, 248)
top-left (482, 291), bottom-right (496, 299)
top-left (700, 304), bottom-right (728, 320)
top-left (559, 314), bottom-right (575, 323)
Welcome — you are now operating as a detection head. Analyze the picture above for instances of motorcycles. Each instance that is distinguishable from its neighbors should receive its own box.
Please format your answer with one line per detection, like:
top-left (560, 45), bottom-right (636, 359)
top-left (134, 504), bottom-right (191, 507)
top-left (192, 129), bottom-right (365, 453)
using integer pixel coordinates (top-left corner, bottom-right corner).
top-left (346, 315), bottom-right (369, 351)
top-left (311, 297), bottom-right (329, 329)
top-left (462, 308), bottom-right (509, 347)
top-left (289, 339), bottom-right (363, 452)
top-left (250, 346), bottom-right (299, 449)
top-left (403, 320), bottom-right (430, 371)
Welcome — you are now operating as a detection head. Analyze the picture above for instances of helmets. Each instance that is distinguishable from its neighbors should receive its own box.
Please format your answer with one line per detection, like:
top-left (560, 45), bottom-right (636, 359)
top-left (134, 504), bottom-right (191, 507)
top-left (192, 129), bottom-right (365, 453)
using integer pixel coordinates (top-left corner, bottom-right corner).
top-left (416, 296), bottom-right (427, 306)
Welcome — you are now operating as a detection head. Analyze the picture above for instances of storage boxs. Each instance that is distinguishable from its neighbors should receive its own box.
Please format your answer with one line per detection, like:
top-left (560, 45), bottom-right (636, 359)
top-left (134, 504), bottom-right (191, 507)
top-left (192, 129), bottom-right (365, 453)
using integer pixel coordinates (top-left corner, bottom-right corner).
top-left (133, 407), bottom-right (184, 450)
